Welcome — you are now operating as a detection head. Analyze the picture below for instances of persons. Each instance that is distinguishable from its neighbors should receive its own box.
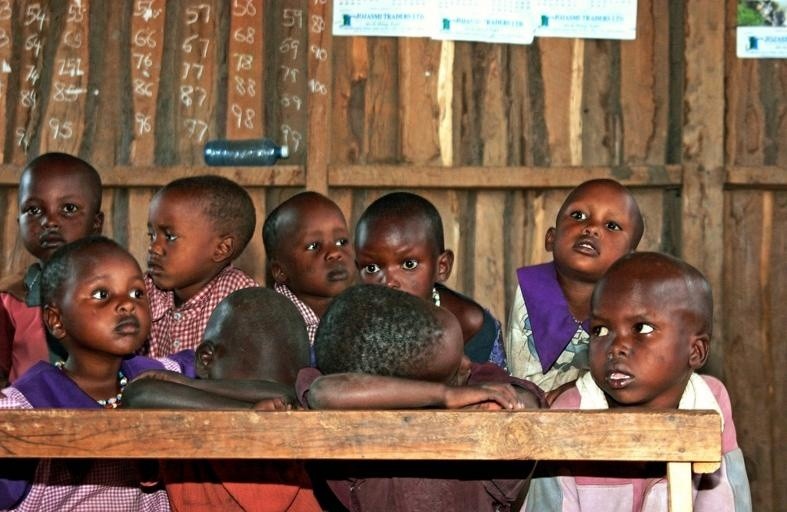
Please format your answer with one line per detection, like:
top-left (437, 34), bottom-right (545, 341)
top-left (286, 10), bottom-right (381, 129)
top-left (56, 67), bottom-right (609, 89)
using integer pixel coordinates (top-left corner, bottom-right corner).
top-left (511, 250), bottom-right (756, 512)
top-left (289, 282), bottom-right (549, 512)
top-left (262, 190), bottom-right (357, 351)
top-left (0, 235), bottom-right (200, 512)
top-left (119, 285), bottom-right (324, 512)
top-left (1, 150), bottom-right (105, 390)
top-left (141, 172), bottom-right (260, 360)
top-left (351, 190), bottom-right (510, 377)
top-left (505, 176), bottom-right (646, 409)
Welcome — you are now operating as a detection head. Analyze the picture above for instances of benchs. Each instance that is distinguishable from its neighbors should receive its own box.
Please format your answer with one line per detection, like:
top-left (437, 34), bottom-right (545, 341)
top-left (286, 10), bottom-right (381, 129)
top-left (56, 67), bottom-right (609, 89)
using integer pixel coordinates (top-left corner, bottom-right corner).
top-left (0, 407), bottom-right (724, 512)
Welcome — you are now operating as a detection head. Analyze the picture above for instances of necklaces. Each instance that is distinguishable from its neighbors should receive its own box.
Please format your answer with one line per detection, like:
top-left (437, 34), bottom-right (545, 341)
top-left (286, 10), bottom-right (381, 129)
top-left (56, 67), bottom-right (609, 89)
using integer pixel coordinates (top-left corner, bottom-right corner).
top-left (53, 359), bottom-right (128, 408)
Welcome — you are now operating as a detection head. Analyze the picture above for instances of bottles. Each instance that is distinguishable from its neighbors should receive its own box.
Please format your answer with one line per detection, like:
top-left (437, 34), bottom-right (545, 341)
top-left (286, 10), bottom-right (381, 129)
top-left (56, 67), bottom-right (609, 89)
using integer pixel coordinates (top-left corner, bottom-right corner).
top-left (202, 138), bottom-right (290, 166)
